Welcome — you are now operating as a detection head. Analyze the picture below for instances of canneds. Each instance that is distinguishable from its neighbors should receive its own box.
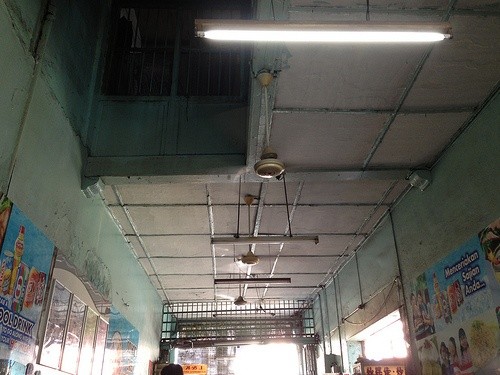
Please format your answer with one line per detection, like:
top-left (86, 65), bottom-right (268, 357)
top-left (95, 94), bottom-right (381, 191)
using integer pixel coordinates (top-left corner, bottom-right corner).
top-left (431, 279), bottom-right (464, 320)
top-left (0, 250), bottom-right (46, 314)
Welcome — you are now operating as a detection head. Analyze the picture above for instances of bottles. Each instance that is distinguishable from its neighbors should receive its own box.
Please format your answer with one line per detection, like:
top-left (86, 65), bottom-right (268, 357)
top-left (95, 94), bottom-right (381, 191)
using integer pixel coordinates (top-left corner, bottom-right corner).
top-left (432, 274), bottom-right (444, 313)
top-left (6, 225), bottom-right (26, 296)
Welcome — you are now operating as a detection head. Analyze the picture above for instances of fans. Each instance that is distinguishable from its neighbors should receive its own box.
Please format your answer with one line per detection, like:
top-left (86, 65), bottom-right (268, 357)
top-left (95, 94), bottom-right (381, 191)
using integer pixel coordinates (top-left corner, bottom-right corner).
top-left (241, 194), bottom-right (260, 266)
top-left (253, 68), bottom-right (286, 179)
top-left (234, 268), bottom-right (248, 306)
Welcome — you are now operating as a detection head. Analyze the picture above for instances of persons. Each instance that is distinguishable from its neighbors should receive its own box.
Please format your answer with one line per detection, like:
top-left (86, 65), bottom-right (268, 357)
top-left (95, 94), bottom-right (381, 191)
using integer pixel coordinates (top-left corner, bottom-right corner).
top-left (439, 327), bottom-right (475, 375)
top-left (161, 363), bottom-right (183, 375)
top-left (410, 289), bottom-right (436, 341)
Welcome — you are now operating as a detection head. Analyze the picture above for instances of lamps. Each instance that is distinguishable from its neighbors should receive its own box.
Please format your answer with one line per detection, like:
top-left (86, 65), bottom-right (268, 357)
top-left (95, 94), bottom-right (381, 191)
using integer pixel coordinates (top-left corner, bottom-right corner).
top-left (214, 278), bottom-right (291, 284)
top-left (210, 235), bottom-right (320, 246)
top-left (194, 17), bottom-right (454, 44)
